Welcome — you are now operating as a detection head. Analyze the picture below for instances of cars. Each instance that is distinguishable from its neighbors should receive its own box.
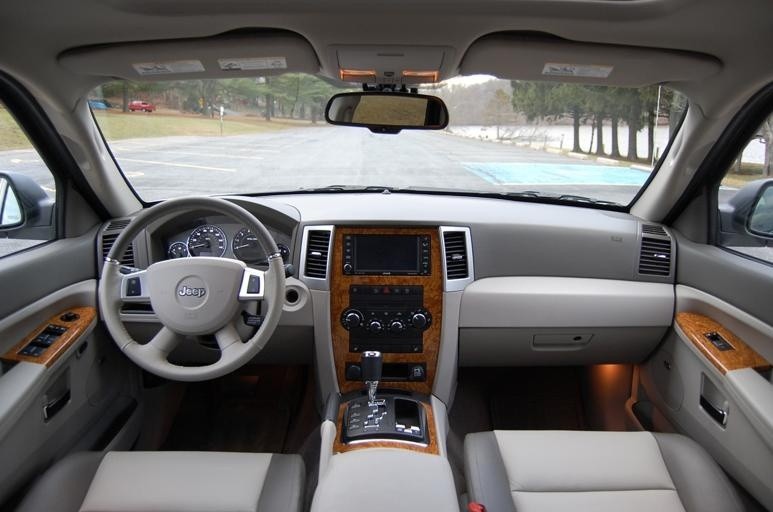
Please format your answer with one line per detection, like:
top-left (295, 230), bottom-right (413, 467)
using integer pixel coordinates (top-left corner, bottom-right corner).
top-left (90, 98), bottom-right (111, 109)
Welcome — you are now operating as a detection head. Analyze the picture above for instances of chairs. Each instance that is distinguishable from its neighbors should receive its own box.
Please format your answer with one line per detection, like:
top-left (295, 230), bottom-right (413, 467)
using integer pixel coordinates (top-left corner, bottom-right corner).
top-left (15, 447), bottom-right (313, 511)
top-left (459, 428), bottom-right (751, 510)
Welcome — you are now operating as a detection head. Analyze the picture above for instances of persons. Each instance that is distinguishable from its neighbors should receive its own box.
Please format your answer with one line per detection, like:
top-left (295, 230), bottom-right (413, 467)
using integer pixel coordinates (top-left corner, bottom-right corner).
top-left (207, 95), bottom-right (220, 118)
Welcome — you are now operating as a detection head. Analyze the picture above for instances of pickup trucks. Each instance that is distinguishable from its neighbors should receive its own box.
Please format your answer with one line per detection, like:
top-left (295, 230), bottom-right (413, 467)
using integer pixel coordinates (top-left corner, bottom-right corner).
top-left (128, 101), bottom-right (155, 112)
